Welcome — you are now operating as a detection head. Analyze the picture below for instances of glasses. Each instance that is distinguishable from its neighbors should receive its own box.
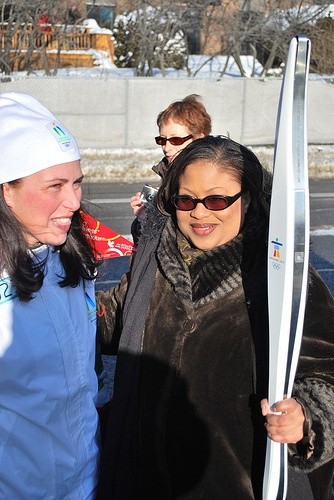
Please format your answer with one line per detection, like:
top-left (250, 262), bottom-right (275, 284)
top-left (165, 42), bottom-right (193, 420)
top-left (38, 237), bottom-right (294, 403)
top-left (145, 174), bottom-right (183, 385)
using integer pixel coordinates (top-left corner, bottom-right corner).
top-left (172, 190), bottom-right (245, 211)
top-left (155, 134), bottom-right (193, 147)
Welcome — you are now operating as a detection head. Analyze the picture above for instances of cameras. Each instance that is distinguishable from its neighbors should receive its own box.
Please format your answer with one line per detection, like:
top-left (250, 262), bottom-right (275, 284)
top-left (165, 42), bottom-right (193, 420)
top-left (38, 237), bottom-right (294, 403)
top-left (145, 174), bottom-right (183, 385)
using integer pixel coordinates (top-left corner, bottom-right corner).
top-left (138, 185), bottom-right (158, 207)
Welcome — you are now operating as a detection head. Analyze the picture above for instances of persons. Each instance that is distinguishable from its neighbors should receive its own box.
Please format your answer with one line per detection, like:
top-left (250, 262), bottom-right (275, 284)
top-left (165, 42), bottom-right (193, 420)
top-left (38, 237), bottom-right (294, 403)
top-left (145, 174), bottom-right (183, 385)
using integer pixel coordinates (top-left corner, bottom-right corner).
top-left (94, 131), bottom-right (334, 500)
top-left (130, 93), bottom-right (212, 217)
top-left (0, 92), bottom-right (100, 500)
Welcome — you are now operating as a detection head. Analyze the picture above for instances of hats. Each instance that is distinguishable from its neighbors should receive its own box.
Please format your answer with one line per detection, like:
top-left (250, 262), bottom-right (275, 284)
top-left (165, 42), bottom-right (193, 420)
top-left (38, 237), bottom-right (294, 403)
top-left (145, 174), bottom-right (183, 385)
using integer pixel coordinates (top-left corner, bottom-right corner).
top-left (0, 91), bottom-right (82, 186)
